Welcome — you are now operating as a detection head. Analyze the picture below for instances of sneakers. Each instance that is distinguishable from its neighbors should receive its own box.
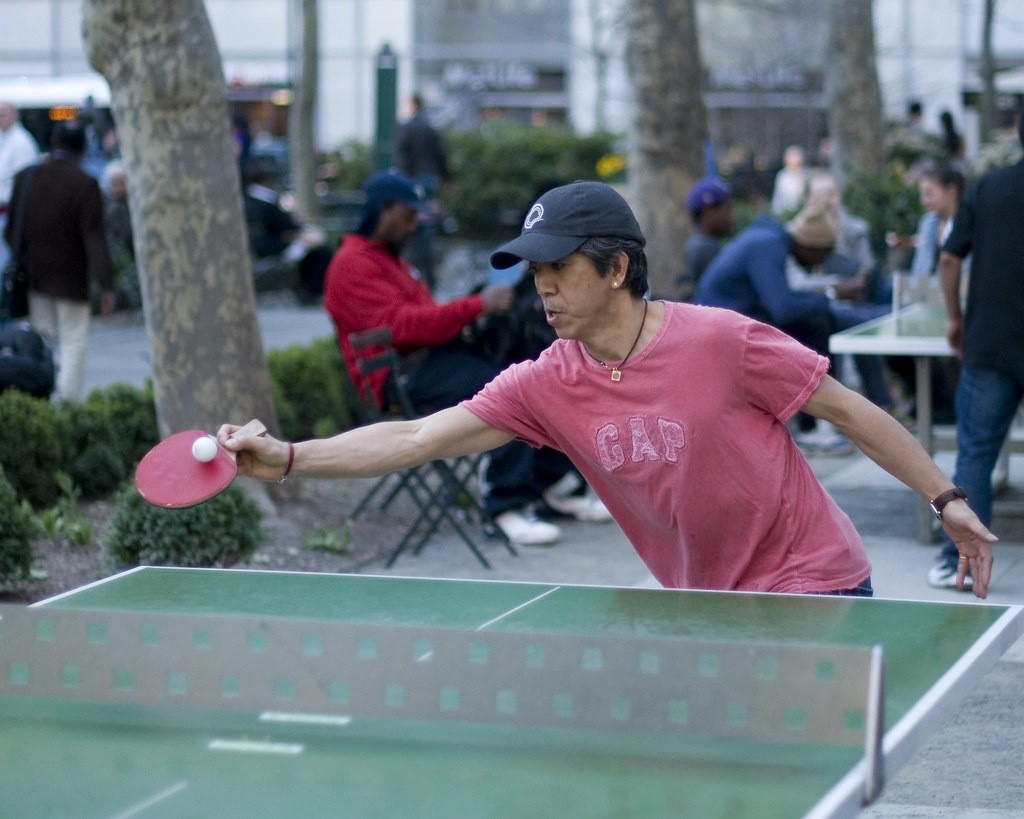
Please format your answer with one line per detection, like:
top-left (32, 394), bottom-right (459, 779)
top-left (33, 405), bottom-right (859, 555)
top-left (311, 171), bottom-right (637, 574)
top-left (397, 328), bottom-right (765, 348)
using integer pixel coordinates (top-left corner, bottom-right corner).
top-left (486, 503), bottom-right (558, 543)
top-left (545, 480), bottom-right (610, 521)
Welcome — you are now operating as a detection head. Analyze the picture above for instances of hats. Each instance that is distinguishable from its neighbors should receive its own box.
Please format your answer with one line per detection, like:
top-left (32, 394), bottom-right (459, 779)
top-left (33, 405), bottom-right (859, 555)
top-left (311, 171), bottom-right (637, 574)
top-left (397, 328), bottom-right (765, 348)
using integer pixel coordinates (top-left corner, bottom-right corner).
top-left (687, 177), bottom-right (729, 209)
top-left (367, 168), bottom-right (434, 214)
top-left (788, 205), bottom-right (837, 248)
top-left (490, 180), bottom-right (647, 271)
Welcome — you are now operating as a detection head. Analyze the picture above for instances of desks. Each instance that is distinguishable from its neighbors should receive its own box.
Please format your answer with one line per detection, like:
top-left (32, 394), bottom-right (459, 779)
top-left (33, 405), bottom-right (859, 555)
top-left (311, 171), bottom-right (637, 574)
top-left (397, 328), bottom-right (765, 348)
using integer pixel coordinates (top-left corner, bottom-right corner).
top-left (0, 564), bottom-right (1024, 819)
top-left (831, 269), bottom-right (1024, 539)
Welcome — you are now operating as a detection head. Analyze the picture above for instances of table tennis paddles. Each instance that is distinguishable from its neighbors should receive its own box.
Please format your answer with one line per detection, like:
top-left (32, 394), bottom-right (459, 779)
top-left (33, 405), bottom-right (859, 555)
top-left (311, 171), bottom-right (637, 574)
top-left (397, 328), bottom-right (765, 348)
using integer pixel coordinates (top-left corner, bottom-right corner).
top-left (134, 417), bottom-right (268, 509)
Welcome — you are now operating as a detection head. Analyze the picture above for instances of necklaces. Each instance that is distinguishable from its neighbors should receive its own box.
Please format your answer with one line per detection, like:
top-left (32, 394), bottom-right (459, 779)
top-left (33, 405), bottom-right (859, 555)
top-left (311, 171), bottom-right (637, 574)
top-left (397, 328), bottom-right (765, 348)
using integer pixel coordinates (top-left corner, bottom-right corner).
top-left (582, 297), bottom-right (648, 381)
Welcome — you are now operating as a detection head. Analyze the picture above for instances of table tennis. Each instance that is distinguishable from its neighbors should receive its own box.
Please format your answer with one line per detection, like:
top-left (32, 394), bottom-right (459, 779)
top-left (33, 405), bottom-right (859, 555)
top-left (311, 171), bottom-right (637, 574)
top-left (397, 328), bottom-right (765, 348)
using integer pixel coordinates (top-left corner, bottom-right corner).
top-left (193, 436), bottom-right (217, 462)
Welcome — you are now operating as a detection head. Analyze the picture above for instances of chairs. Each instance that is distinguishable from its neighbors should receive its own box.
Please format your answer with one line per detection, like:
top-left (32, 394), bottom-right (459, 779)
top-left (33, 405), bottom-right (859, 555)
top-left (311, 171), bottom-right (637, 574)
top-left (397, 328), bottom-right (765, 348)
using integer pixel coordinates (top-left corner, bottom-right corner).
top-left (337, 332), bottom-right (520, 570)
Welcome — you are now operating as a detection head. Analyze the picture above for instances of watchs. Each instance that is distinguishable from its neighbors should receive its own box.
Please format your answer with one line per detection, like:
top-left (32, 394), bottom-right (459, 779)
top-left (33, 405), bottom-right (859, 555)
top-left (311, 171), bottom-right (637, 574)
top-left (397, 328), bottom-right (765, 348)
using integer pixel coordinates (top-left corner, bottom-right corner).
top-left (928, 486), bottom-right (969, 520)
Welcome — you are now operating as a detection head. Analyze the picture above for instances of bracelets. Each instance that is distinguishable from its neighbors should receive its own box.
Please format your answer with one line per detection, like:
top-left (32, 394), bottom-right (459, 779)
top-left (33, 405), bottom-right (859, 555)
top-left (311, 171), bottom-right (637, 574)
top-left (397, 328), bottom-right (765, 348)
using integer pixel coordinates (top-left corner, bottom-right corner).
top-left (277, 441), bottom-right (295, 485)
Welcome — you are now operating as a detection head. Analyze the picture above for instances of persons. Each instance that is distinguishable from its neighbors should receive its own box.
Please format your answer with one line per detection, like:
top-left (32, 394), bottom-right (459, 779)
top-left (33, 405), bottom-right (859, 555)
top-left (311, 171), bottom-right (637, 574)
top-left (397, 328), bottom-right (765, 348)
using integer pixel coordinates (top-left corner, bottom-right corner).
top-left (691, 176), bottom-right (867, 457)
top-left (938, 111), bottom-right (966, 165)
top-left (232, 109), bottom-right (249, 183)
top-left (675, 145), bottom-right (731, 303)
top-left (907, 101), bottom-right (928, 133)
top-left (99, 160), bottom-right (136, 269)
top-left (3, 119), bottom-right (119, 403)
top-left (927, 96), bottom-right (1024, 593)
top-left (772, 147), bottom-right (807, 217)
top-left (217, 180), bottom-right (999, 599)
top-left (0, 100), bottom-right (43, 360)
top-left (884, 166), bottom-right (972, 426)
top-left (783, 171), bottom-right (895, 412)
top-left (393, 92), bottom-right (451, 293)
top-left (322, 169), bottom-right (614, 546)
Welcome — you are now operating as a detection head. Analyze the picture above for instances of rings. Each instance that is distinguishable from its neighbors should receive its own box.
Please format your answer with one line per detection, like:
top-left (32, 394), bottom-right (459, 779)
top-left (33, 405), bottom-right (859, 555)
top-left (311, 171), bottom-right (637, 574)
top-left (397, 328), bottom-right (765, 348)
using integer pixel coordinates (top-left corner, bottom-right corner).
top-left (959, 556), bottom-right (969, 559)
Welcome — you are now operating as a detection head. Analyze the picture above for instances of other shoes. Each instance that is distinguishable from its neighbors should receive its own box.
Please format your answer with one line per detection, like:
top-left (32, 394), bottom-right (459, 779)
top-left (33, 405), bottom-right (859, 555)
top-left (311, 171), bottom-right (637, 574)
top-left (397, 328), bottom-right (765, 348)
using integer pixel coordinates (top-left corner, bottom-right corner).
top-left (929, 562), bottom-right (974, 589)
